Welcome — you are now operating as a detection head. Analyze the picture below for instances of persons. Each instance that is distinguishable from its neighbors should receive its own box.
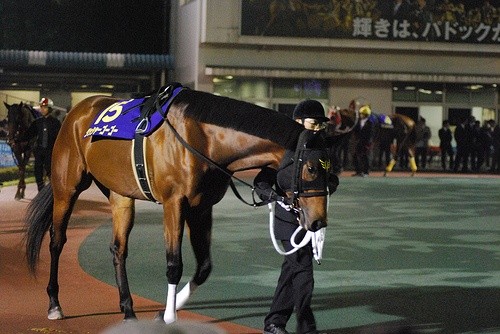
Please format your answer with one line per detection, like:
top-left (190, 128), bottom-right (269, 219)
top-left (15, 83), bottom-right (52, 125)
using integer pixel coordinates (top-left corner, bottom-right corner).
top-left (351, 105), bottom-right (500, 178)
top-left (6, 97), bottom-right (62, 193)
top-left (253, 99), bottom-right (340, 334)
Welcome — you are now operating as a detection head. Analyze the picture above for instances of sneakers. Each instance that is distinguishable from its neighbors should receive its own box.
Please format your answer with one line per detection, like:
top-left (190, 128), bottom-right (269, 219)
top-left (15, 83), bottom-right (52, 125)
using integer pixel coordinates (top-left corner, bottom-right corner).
top-left (352, 172), bottom-right (368, 176)
top-left (263, 324), bottom-right (328, 334)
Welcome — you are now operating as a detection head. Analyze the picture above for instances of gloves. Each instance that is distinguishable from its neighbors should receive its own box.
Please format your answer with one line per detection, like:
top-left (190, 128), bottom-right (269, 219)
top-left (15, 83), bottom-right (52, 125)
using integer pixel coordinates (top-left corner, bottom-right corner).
top-left (277, 197), bottom-right (294, 211)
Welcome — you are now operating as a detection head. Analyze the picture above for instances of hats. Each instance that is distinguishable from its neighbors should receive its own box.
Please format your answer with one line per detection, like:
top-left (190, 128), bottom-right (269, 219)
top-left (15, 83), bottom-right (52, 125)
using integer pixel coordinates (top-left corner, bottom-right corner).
top-left (359, 106), bottom-right (372, 114)
top-left (39, 98), bottom-right (53, 107)
top-left (293, 98), bottom-right (330, 121)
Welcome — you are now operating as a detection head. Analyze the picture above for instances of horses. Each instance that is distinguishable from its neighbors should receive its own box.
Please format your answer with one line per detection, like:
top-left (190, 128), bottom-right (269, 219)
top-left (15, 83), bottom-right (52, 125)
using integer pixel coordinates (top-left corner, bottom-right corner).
top-left (2, 101), bottom-right (46, 200)
top-left (326, 103), bottom-right (419, 177)
top-left (18, 86), bottom-right (347, 327)
